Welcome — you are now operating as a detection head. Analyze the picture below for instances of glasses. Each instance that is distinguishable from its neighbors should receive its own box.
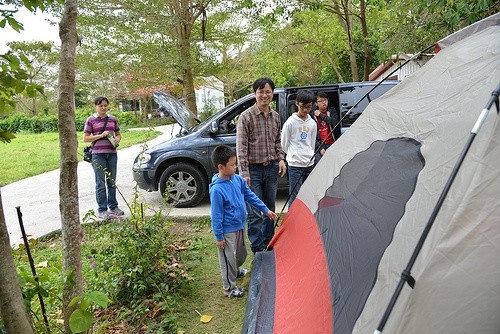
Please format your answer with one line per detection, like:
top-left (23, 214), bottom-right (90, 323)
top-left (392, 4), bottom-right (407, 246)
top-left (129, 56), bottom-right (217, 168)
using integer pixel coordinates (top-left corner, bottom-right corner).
top-left (298, 103), bottom-right (312, 109)
top-left (318, 98), bottom-right (328, 102)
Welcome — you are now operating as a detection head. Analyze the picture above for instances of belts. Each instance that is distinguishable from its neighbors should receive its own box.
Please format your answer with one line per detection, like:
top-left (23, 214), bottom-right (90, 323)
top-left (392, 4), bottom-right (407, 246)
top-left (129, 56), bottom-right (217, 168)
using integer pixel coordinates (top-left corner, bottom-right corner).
top-left (253, 160), bottom-right (275, 166)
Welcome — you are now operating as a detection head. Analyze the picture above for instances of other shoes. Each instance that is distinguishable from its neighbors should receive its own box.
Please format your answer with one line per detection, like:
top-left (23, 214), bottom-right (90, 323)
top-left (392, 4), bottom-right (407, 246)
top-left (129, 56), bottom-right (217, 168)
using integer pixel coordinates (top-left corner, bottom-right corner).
top-left (224, 287), bottom-right (243, 297)
top-left (239, 268), bottom-right (250, 276)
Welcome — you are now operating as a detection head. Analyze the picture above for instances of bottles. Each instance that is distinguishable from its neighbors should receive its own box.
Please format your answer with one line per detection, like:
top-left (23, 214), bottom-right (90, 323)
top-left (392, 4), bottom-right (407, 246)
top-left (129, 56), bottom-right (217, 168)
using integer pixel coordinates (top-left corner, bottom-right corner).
top-left (106, 133), bottom-right (119, 148)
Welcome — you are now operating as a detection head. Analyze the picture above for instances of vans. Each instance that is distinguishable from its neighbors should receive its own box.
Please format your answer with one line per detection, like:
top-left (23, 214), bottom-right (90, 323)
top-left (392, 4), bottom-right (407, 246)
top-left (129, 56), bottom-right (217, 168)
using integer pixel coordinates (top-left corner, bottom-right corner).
top-left (132, 80), bottom-right (402, 207)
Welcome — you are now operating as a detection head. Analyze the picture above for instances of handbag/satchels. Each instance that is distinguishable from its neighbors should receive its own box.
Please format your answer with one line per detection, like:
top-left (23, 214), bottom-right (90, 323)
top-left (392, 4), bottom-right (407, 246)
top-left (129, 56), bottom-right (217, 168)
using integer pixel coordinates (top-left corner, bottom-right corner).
top-left (84, 145), bottom-right (93, 163)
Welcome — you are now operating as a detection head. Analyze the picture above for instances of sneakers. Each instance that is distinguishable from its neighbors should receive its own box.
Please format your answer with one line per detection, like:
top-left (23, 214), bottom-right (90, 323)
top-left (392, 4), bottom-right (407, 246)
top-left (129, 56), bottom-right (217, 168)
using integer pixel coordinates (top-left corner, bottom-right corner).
top-left (111, 208), bottom-right (124, 215)
top-left (99, 210), bottom-right (114, 219)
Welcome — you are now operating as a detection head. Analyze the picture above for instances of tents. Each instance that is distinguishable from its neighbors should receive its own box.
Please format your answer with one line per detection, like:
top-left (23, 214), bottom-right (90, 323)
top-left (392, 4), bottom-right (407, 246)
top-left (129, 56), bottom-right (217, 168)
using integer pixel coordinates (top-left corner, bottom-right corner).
top-left (240, 10), bottom-right (500, 334)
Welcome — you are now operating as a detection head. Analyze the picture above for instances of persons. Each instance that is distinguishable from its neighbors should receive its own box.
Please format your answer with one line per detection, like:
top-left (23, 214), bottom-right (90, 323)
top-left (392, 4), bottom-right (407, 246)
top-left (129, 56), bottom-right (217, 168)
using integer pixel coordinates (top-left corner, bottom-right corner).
top-left (310, 92), bottom-right (341, 164)
top-left (280, 89), bottom-right (317, 205)
top-left (235, 78), bottom-right (287, 254)
top-left (209, 145), bottom-right (278, 298)
top-left (84, 97), bottom-right (124, 218)
